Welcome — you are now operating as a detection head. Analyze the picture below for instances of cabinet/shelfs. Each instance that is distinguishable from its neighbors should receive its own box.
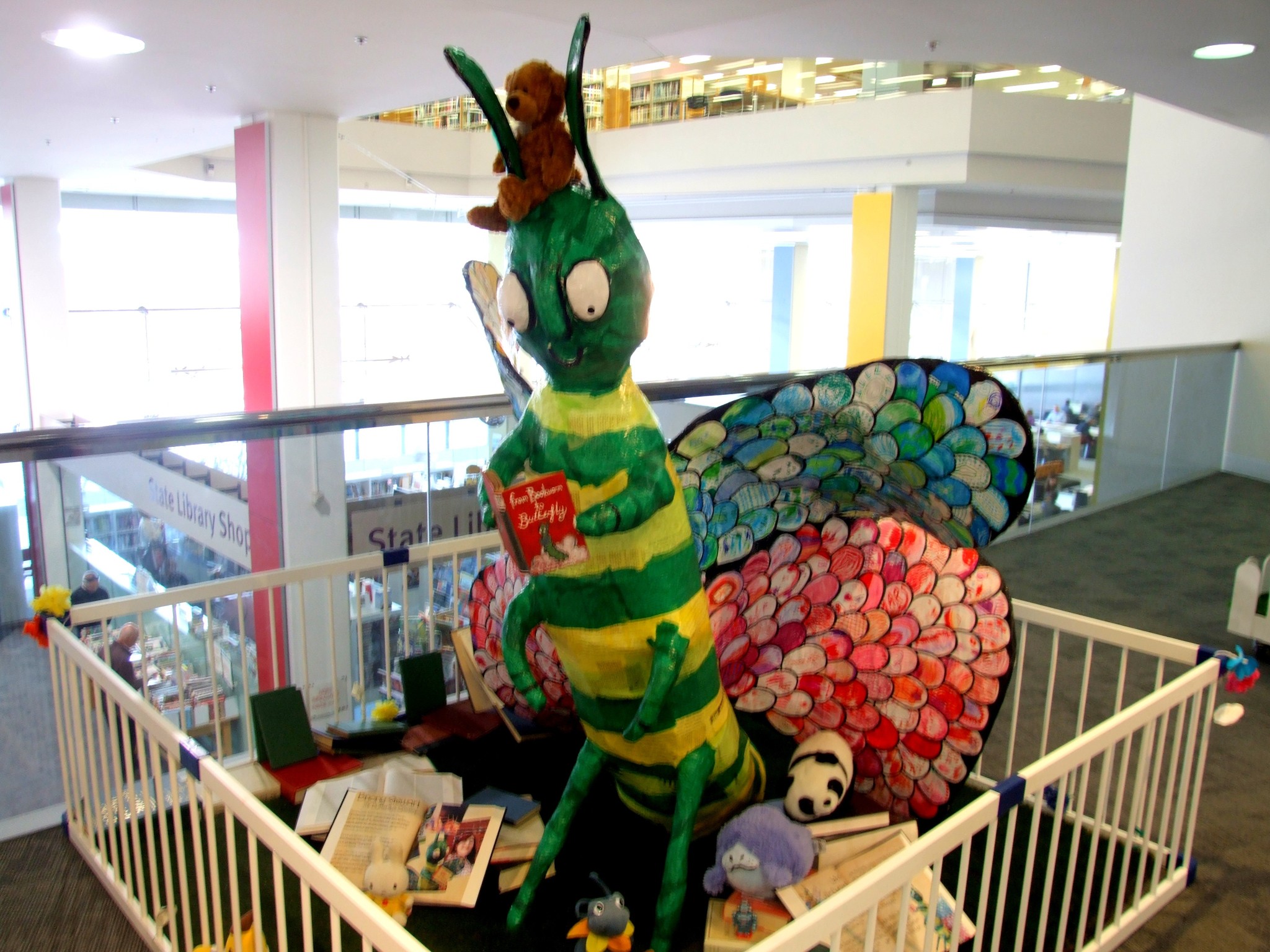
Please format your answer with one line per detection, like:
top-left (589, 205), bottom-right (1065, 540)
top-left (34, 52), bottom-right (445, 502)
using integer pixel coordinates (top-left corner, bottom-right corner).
top-left (81, 501), bottom-right (165, 569)
top-left (363, 71), bottom-right (704, 136)
top-left (346, 464), bottom-right (458, 510)
top-left (378, 547), bottom-right (502, 712)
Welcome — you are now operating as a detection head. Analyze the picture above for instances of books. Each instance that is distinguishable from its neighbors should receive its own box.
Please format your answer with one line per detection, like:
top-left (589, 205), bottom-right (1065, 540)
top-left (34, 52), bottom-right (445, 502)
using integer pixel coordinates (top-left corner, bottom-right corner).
top-left (199, 626), bottom-right (559, 911)
top-left (704, 812), bottom-right (982, 952)
top-left (93, 619), bottom-right (229, 735)
top-left (413, 79), bottom-right (680, 126)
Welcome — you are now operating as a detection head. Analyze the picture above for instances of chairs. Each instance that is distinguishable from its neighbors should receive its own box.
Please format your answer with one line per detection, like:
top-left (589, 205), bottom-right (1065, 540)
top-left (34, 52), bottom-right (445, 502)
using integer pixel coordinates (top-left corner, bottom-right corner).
top-left (719, 90), bottom-right (752, 118)
top-left (686, 95), bottom-right (716, 119)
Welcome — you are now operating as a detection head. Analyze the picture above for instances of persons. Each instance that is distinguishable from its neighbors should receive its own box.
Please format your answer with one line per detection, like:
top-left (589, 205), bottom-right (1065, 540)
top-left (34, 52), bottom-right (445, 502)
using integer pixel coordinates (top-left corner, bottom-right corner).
top-left (130, 506), bottom-right (186, 590)
top-left (67, 570), bottom-right (111, 637)
top-left (1021, 399), bottom-right (1100, 523)
top-left (91, 621), bottom-right (150, 788)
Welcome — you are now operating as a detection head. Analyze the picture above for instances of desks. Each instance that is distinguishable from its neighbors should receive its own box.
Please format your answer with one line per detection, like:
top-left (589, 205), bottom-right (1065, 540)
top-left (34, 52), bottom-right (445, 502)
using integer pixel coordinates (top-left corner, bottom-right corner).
top-left (83, 659), bottom-right (241, 778)
top-left (680, 92), bottom-right (808, 121)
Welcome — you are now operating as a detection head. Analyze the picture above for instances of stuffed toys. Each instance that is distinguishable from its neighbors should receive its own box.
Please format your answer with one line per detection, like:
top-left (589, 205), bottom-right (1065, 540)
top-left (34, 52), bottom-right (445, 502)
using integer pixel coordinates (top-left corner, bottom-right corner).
top-left (189, 908), bottom-right (269, 952)
top-left (463, 59), bottom-right (588, 233)
top-left (785, 730), bottom-right (857, 824)
top-left (360, 838), bottom-right (418, 927)
top-left (562, 875), bottom-right (635, 952)
top-left (697, 797), bottom-right (819, 898)
